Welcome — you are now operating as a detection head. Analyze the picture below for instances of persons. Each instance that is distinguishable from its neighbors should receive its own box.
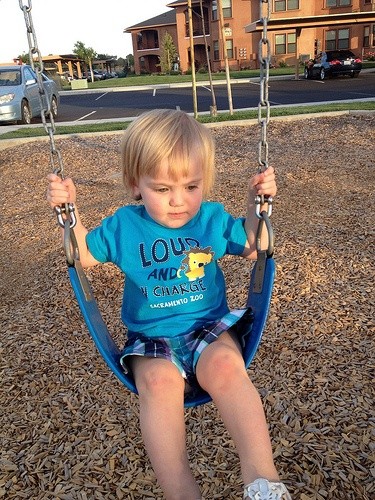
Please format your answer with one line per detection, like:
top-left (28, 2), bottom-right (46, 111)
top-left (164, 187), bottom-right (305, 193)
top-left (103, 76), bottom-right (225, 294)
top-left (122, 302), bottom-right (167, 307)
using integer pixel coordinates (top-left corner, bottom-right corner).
top-left (46, 108), bottom-right (291, 500)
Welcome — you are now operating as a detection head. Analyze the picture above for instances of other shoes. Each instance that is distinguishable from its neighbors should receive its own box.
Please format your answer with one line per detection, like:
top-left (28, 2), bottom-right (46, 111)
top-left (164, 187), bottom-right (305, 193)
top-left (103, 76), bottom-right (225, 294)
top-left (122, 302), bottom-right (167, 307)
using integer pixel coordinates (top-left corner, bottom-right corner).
top-left (243, 477), bottom-right (292, 500)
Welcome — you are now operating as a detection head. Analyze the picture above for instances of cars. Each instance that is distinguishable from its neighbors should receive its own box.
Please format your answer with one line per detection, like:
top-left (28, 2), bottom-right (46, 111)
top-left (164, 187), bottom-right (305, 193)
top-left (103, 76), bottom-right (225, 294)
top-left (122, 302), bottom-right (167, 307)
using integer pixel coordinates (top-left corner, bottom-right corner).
top-left (0, 65), bottom-right (60, 125)
top-left (304, 49), bottom-right (362, 81)
top-left (64, 70), bottom-right (117, 82)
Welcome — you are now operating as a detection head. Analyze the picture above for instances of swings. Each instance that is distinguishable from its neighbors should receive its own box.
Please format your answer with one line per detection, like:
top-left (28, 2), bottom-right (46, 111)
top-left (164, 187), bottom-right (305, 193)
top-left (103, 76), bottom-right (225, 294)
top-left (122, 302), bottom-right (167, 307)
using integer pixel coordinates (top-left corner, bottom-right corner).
top-left (17, 0), bottom-right (275, 408)
